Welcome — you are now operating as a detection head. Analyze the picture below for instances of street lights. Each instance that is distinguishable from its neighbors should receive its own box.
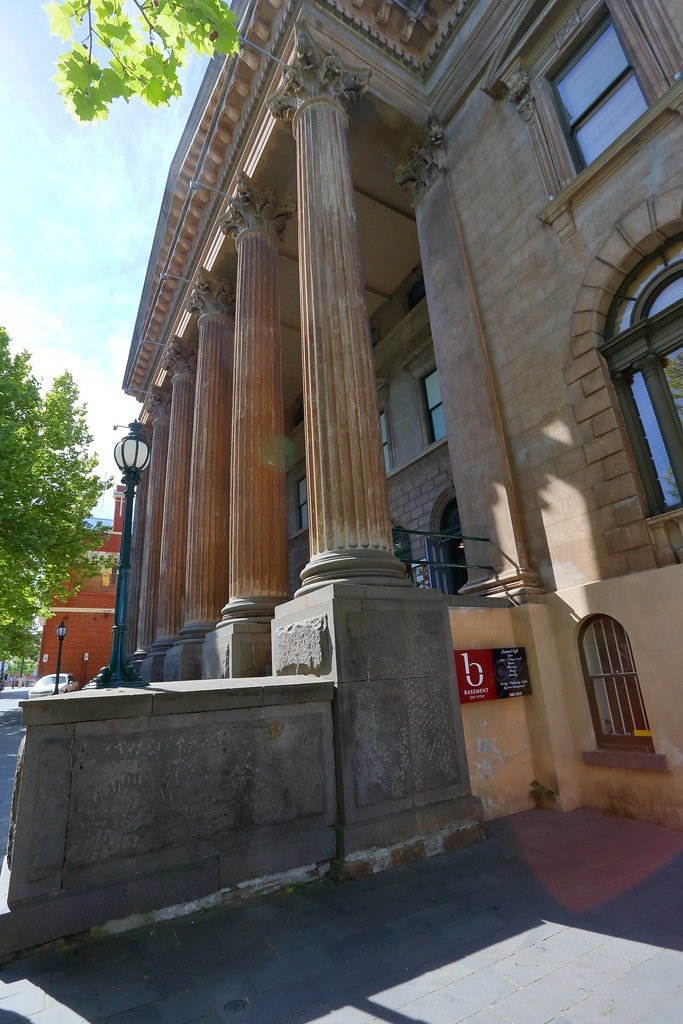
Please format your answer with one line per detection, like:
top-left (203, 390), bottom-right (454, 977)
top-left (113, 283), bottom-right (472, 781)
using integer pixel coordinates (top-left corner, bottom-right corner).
top-left (52, 620), bottom-right (67, 695)
top-left (95, 419), bottom-right (152, 687)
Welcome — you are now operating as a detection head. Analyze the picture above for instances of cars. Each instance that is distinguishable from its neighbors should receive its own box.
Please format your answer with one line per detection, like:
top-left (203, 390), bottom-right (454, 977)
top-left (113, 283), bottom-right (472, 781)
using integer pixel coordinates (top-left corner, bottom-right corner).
top-left (28, 674), bottom-right (79, 699)
